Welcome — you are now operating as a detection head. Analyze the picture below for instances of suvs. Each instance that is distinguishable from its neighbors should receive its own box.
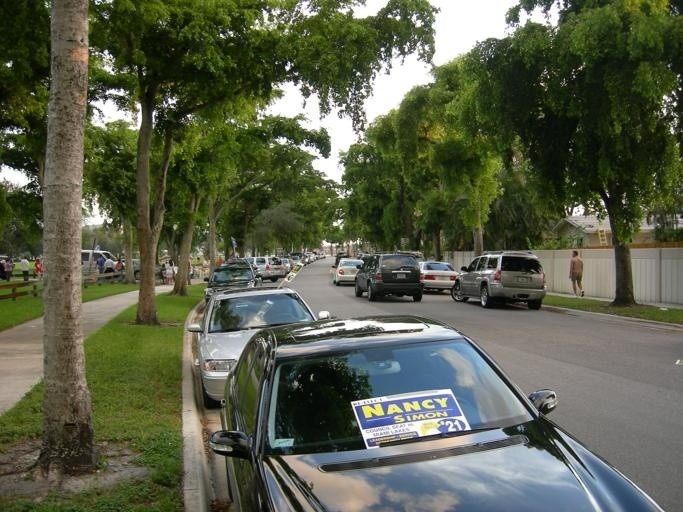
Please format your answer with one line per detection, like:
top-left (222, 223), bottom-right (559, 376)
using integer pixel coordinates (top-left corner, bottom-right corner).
top-left (452, 252), bottom-right (547, 309)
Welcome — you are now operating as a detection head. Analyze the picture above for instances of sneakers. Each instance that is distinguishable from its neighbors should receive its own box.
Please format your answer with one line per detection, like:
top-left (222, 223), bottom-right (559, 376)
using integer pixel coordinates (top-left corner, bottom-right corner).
top-left (580, 290), bottom-right (584, 297)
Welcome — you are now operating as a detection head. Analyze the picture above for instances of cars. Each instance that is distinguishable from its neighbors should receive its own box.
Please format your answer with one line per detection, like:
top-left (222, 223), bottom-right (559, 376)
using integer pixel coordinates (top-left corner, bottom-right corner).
top-left (187, 286), bottom-right (332, 412)
top-left (208, 315), bottom-right (665, 512)
top-left (331, 250), bottom-right (459, 302)
top-left (203, 251), bottom-right (328, 302)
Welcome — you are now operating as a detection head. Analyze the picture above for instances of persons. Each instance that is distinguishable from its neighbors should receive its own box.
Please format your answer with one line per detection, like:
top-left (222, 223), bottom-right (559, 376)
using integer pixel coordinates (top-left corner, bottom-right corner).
top-left (34, 258), bottom-right (42, 281)
top-left (3, 259), bottom-right (14, 282)
top-left (19, 255), bottom-right (30, 281)
top-left (568, 250), bottom-right (583, 297)
top-left (92, 254), bottom-right (222, 286)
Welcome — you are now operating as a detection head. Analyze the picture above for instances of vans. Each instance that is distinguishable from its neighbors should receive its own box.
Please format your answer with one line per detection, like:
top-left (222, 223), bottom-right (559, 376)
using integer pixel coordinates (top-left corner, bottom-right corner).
top-left (82, 249), bottom-right (141, 281)
